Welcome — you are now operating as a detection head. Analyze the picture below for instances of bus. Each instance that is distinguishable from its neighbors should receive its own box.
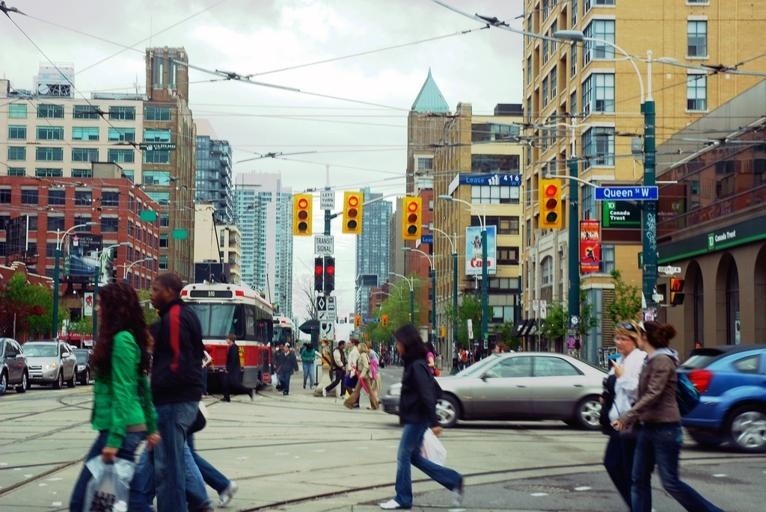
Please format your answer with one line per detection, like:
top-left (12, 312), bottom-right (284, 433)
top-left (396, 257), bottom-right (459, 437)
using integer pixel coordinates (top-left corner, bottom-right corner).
top-left (272, 314), bottom-right (296, 348)
top-left (179, 278), bottom-right (277, 394)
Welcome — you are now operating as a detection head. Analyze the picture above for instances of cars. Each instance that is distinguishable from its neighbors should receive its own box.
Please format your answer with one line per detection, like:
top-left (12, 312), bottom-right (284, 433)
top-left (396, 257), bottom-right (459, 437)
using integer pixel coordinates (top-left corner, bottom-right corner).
top-left (72, 347), bottom-right (94, 386)
top-left (0, 336), bottom-right (31, 395)
top-left (378, 350), bottom-right (608, 431)
top-left (674, 340), bottom-right (766, 453)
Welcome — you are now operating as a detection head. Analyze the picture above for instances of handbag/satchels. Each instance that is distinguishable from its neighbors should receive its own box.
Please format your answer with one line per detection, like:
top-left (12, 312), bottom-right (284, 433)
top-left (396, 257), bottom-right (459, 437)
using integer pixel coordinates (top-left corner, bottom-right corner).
top-left (434, 367), bottom-right (440, 376)
top-left (599, 375), bottom-right (616, 434)
top-left (675, 366), bottom-right (699, 415)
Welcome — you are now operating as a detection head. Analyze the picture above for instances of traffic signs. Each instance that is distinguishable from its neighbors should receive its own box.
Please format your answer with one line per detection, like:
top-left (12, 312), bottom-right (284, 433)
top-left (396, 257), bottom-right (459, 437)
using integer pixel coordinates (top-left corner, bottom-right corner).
top-left (594, 186), bottom-right (659, 201)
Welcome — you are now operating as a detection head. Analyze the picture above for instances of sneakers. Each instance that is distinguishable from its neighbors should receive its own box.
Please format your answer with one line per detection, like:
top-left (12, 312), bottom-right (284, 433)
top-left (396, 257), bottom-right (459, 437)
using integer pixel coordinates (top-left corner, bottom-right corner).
top-left (452, 479), bottom-right (463, 507)
top-left (218, 481), bottom-right (238, 508)
top-left (381, 498), bottom-right (401, 510)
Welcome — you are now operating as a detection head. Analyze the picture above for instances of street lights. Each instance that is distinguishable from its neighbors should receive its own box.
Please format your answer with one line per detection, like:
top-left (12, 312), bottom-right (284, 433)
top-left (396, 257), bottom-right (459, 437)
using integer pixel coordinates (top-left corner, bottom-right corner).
top-left (422, 223), bottom-right (459, 340)
top-left (400, 246), bottom-right (437, 350)
top-left (543, 171), bottom-right (597, 191)
top-left (369, 270), bottom-right (416, 323)
top-left (552, 28), bottom-right (666, 318)
top-left (435, 193), bottom-right (491, 360)
top-left (123, 256), bottom-right (154, 283)
top-left (52, 218), bottom-right (97, 338)
top-left (91, 238), bottom-right (135, 341)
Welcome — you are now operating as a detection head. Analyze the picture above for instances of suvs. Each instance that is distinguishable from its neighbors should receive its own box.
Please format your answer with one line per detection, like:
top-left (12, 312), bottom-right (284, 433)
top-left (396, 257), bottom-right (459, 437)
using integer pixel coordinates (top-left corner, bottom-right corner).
top-left (19, 338), bottom-right (76, 390)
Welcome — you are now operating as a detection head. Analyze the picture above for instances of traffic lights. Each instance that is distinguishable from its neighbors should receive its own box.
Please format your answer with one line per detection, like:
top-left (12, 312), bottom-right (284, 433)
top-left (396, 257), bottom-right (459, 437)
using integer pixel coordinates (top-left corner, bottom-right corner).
top-left (670, 277), bottom-right (686, 307)
top-left (324, 257), bottom-right (335, 291)
top-left (539, 180), bottom-right (562, 229)
top-left (382, 315), bottom-right (388, 327)
top-left (342, 191), bottom-right (363, 235)
top-left (401, 196), bottom-right (423, 241)
top-left (355, 315), bottom-right (361, 327)
top-left (292, 192), bottom-right (312, 236)
top-left (315, 258), bottom-right (323, 291)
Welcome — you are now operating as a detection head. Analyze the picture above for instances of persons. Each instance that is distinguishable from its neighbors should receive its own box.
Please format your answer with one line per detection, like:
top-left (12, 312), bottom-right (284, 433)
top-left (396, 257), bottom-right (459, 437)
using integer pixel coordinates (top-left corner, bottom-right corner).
top-left (610, 320), bottom-right (725, 511)
top-left (222, 334), bottom-right (253, 402)
top-left (322, 339), bottom-right (378, 408)
top-left (424, 342), bottom-right (436, 376)
top-left (299, 343), bottom-right (316, 389)
top-left (220, 330), bottom-right (524, 410)
top-left (602, 318), bottom-right (656, 511)
top-left (272, 341), bottom-right (298, 395)
top-left (128, 266), bottom-right (238, 512)
top-left (377, 324), bottom-right (464, 509)
top-left (66, 280), bottom-right (152, 511)
top-left (456, 342), bottom-right (504, 372)
